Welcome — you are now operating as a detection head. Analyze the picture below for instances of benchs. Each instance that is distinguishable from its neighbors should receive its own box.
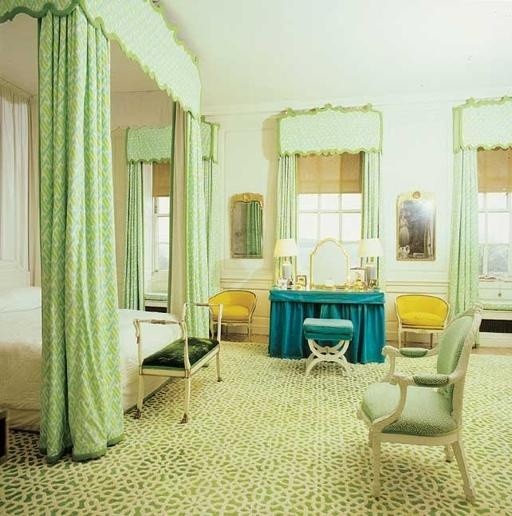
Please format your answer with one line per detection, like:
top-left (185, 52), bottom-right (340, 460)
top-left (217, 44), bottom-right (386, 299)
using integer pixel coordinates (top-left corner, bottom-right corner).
top-left (132, 303), bottom-right (225, 425)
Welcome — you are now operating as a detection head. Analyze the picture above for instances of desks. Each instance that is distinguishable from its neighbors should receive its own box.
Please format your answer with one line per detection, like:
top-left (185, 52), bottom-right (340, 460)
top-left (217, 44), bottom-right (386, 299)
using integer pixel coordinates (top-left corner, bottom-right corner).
top-left (270, 287), bottom-right (385, 363)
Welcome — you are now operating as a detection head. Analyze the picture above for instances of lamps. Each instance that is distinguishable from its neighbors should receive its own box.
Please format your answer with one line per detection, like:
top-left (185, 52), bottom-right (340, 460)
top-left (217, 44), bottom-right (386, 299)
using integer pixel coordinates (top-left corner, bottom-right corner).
top-left (359, 237), bottom-right (384, 286)
top-left (271, 237), bottom-right (299, 286)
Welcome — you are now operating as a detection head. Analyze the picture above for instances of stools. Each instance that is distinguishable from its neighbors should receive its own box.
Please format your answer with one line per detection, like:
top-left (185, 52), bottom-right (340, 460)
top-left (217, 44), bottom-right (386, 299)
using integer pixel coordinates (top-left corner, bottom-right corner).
top-left (298, 319), bottom-right (355, 380)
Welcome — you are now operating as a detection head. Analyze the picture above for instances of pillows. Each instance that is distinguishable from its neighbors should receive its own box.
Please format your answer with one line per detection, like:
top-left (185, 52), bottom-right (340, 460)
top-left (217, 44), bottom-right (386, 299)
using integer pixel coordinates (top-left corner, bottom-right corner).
top-left (0, 287), bottom-right (41, 313)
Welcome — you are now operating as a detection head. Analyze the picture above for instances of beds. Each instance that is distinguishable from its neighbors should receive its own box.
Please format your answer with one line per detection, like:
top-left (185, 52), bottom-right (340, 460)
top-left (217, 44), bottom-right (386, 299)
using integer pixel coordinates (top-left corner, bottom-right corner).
top-left (0, 282), bottom-right (183, 437)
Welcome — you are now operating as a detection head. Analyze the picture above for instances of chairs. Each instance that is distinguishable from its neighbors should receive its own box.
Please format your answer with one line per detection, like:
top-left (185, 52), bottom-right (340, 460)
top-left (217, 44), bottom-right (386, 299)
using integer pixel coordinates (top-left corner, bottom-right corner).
top-left (206, 290), bottom-right (257, 340)
top-left (393, 295), bottom-right (449, 351)
top-left (355, 304), bottom-right (484, 502)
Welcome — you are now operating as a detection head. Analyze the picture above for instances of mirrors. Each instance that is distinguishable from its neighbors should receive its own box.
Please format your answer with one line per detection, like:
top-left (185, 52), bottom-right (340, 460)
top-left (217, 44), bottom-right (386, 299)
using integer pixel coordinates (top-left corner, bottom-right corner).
top-left (396, 189), bottom-right (439, 261)
top-left (228, 193), bottom-right (264, 260)
top-left (293, 152), bottom-right (364, 291)
